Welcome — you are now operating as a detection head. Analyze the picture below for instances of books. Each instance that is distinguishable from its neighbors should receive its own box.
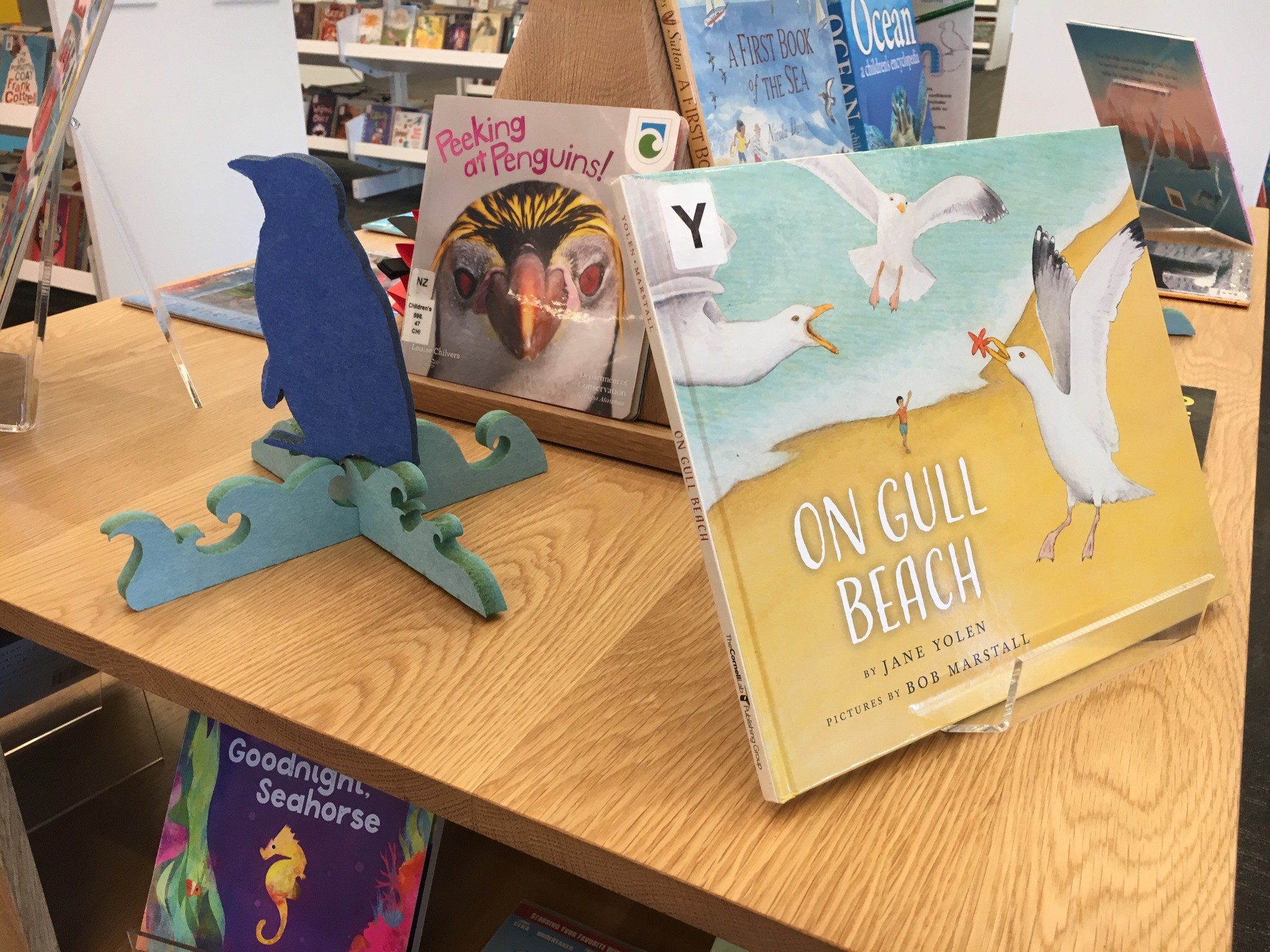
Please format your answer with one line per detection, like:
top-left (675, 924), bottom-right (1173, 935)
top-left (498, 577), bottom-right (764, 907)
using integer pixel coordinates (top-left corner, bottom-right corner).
top-left (1145, 238), bottom-right (1253, 307)
top-left (656, 0), bottom-right (854, 170)
top-left (361, 211), bottom-right (413, 237)
top-left (400, 94), bottom-right (689, 420)
top-left (141, 710), bottom-right (445, 952)
top-left (120, 262), bottom-right (265, 338)
top-left (301, 90), bottom-right (433, 150)
top-left (0, 148), bottom-right (95, 273)
top-left (610, 122), bottom-right (1234, 805)
top-left (292, 0), bottom-right (524, 53)
top-left (1, 22), bottom-right (61, 108)
top-left (828, 0), bottom-right (936, 153)
top-left (1067, 19), bottom-right (1257, 245)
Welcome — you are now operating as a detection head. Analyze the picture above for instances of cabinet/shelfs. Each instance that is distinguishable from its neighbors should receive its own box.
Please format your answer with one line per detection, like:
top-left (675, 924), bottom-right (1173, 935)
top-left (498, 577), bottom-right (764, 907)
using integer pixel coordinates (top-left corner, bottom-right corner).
top-left (0, 0), bottom-right (308, 299)
top-left (293, 0), bottom-right (527, 201)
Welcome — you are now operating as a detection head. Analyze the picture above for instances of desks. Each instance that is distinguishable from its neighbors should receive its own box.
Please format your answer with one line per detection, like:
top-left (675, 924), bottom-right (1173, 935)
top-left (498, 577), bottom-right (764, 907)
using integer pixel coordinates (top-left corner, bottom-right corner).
top-left (0, 208), bottom-right (1270, 952)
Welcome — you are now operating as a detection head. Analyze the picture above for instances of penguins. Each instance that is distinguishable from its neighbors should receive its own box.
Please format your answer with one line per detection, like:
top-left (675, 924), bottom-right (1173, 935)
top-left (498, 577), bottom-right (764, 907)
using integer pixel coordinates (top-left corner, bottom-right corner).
top-left (224, 148), bottom-right (424, 474)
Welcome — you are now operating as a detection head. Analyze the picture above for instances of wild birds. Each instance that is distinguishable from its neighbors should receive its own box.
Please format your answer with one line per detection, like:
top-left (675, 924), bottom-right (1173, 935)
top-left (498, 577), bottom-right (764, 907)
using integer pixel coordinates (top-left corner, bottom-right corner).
top-left (422, 175), bottom-right (628, 419)
